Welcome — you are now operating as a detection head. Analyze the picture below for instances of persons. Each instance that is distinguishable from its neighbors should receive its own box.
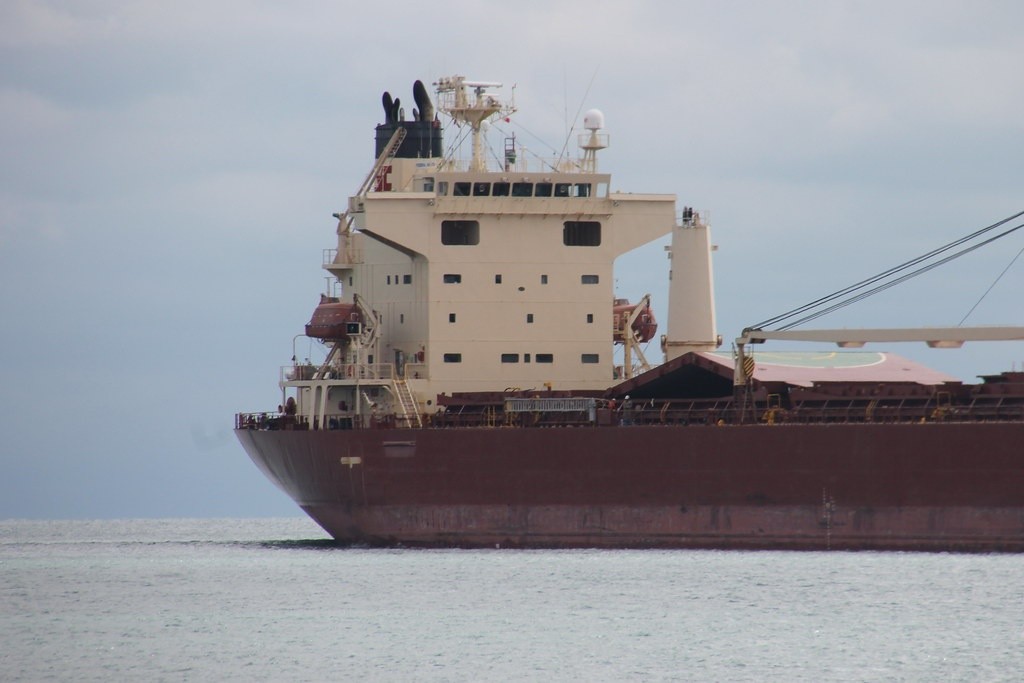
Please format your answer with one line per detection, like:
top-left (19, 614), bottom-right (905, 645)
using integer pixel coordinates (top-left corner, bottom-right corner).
top-left (682, 206), bottom-right (692, 225)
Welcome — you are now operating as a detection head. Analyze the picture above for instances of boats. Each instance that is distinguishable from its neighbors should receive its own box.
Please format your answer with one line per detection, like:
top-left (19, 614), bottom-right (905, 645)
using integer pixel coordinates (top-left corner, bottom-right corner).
top-left (232, 76), bottom-right (1024, 552)
